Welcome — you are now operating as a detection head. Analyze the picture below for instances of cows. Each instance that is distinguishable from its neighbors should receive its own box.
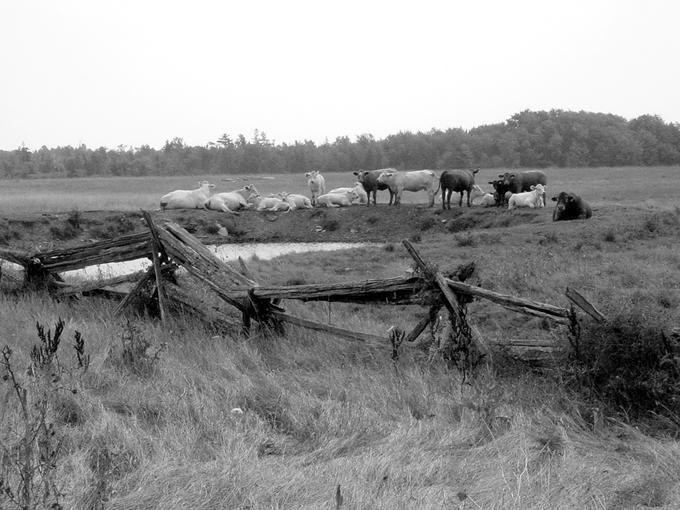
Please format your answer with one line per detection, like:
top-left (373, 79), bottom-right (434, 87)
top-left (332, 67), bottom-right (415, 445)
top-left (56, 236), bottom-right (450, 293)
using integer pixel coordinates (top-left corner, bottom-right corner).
top-left (435, 168), bottom-right (549, 216)
top-left (156, 167), bottom-right (374, 216)
top-left (548, 190), bottom-right (592, 221)
top-left (353, 168), bottom-right (440, 216)
